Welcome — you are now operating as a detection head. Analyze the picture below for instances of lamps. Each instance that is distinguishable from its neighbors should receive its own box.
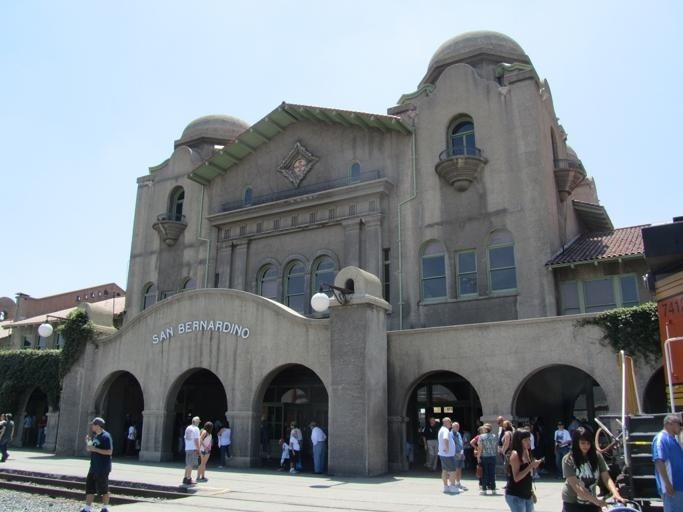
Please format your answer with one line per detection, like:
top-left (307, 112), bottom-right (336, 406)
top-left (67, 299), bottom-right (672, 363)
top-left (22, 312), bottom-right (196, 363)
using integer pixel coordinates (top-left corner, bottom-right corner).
top-left (36, 313), bottom-right (78, 338)
top-left (308, 283), bottom-right (354, 313)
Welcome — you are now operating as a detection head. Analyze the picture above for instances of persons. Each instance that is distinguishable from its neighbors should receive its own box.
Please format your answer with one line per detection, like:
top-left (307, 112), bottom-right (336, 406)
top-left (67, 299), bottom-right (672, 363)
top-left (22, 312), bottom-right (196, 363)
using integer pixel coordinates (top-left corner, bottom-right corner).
top-left (0, 411), bottom-right (46, 462)
top-left (123, 422), bottom-right (137, 457)
top-left (173, 416), bottom-right (230, 483)
top-left (421, 412), bottom-right (681, 511)
top-left (81, 417), bottom-right (114, 511)
top-left (277, 420), bottom-right (327, 473)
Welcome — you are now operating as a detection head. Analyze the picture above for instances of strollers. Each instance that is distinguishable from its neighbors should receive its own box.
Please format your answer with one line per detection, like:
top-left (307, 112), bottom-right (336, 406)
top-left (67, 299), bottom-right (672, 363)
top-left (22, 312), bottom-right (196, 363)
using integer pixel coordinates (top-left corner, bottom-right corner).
top-left (596, 497), bottom-right (643, 512)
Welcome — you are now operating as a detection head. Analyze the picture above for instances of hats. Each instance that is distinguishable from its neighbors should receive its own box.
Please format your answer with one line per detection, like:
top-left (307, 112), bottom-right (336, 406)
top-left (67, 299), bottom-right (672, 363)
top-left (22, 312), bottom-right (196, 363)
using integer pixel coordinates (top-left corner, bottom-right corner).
top-left (289, 420), bottom-right (318, 427)
top-left (89, 418), bottom-right (105, 428)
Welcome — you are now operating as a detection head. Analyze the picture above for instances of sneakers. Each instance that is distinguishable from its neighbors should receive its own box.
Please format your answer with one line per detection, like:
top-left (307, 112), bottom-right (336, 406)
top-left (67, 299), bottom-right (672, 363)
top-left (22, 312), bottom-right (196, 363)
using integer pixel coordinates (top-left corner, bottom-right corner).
top-left (184, 475), bottom-right (210, 484)
top-left (443, 482), bottom-right (469, 493)
top-left (478, 485), bottom-right (499, 495)
top-left (79, 508), bottom-right (91, 512)
top-left (100, 508), bottom-right (109, 512)
